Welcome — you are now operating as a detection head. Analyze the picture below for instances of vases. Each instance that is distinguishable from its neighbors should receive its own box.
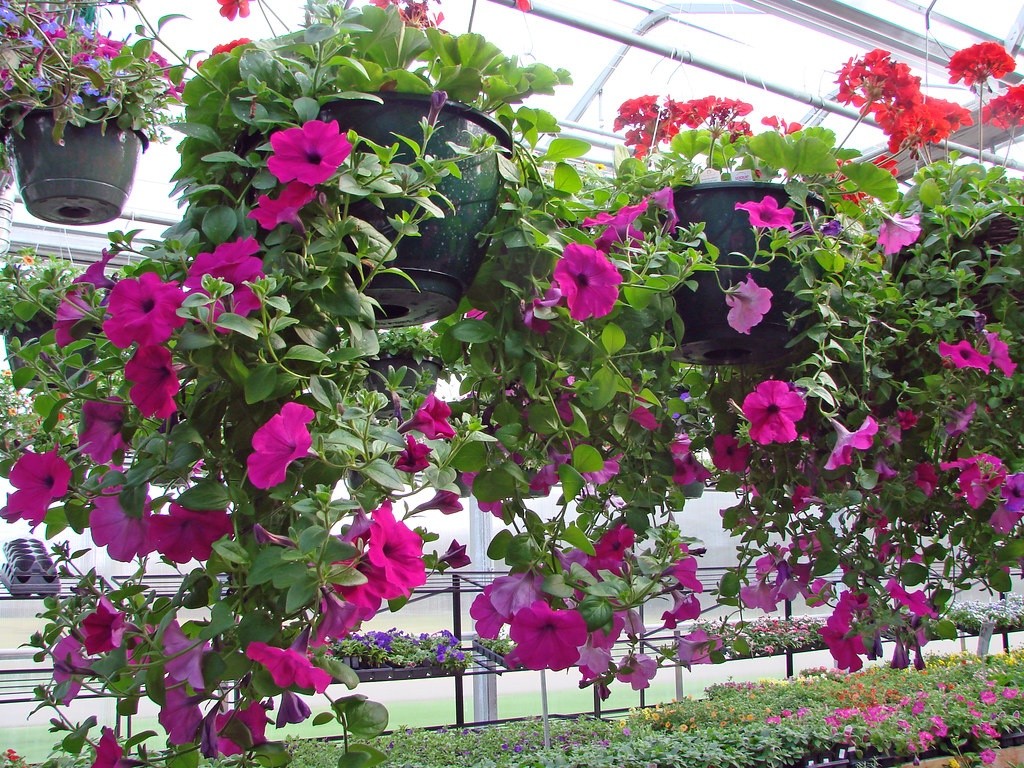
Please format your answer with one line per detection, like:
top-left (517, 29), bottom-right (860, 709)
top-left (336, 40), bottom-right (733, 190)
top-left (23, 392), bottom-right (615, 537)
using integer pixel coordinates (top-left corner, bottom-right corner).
top-left (364, 349), bottom-right (444, 417)
top-left (807, 759), bottom-right (850, 768)
top-left (394, 667), bottom-right (431, 678)
top-left (656, 181), bottom-right (837, 365)
top-left (898, 754), bottom-right (915, 762)
top-left (258, 93), bottom-right (513, 330)
top-left (0, 108), bottom-right (150, 225)
top-left (890, 206), bottom-right (1021, 323)
top-left (868, 757), bottom-right (894, 767)
top-left (353, 665), bottom-right (393, 682)
top-left (424, 663), bottom-right (465, 676)
top-left (919, 750), bottom-right (938, 760)
top-left (473, 640), bottom-right (509, 668)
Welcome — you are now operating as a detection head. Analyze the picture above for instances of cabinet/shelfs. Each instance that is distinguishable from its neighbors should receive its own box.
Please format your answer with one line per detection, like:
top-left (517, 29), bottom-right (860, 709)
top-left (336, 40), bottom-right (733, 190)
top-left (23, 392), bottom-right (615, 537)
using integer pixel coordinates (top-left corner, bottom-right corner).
top-left (0, 564), bottom-right (1024, 768)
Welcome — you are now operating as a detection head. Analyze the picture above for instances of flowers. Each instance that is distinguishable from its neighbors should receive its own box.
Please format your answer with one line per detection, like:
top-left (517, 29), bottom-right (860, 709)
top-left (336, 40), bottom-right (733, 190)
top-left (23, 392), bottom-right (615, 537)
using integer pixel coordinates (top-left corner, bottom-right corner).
top-left (0, 1), bottom-right (1024, 768)
